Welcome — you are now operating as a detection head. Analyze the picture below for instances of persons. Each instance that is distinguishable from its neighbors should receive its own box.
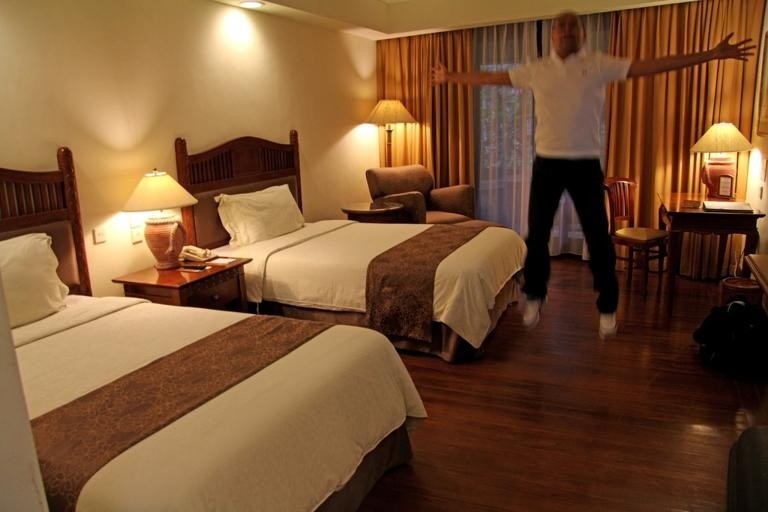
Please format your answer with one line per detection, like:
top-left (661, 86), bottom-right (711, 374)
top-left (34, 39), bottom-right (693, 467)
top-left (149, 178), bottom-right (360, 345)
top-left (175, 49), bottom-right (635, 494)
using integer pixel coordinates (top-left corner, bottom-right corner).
top-left (429, 10), bottom-right (757, 340)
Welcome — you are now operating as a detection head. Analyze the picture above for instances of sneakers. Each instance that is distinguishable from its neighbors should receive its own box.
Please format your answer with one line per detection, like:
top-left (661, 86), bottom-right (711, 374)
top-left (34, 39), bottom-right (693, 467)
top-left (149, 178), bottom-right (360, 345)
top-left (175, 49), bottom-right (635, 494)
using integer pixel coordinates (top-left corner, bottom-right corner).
top-left (522, 300), bottom-right (541, 327)
top-left (599, 312), bottom-right (616, 336)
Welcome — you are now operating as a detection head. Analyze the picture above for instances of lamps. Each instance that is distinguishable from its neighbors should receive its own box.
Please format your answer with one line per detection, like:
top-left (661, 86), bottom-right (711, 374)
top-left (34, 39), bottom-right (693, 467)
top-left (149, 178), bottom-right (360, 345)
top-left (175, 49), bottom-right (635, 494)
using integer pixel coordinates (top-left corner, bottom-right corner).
top-left (687, 120), bottom-right (755, 201)
top-left (118, 166), bottom-right (200, 272)
top-left (362, 99), bottom-right (420, 168)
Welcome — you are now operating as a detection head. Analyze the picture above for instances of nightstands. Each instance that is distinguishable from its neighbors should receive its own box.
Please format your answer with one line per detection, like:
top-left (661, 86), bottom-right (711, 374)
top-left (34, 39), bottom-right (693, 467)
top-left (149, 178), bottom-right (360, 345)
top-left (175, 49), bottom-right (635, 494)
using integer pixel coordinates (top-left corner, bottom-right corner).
top-left (112, 257), bottom-right (253, 314)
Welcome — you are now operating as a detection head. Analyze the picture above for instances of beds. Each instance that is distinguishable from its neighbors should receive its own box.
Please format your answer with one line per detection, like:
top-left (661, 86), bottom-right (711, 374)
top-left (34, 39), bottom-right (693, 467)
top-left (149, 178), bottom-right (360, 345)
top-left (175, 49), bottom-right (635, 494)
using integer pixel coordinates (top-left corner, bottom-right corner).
top-left (172, 128), bottom-right (528, 366)
top-left (0, 144), bottom-right (431, 512)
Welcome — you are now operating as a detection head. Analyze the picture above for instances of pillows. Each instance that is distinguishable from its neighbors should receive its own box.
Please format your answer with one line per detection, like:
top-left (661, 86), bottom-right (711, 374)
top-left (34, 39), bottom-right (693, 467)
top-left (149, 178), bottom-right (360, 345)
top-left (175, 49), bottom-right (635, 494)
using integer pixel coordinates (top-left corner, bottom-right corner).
top-left (0, 230), bottom-right (72, 331)
top-left (213, 183), bottom-right (308, 250)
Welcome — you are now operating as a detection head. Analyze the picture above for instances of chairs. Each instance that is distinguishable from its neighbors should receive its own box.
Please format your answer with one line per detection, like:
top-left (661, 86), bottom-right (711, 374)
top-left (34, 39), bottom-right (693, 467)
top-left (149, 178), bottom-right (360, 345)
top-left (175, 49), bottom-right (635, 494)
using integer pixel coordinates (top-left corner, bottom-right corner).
top-left (365, 163), bottom-right (476, 225)
top-left (601, 176), bottom-right (670, 298)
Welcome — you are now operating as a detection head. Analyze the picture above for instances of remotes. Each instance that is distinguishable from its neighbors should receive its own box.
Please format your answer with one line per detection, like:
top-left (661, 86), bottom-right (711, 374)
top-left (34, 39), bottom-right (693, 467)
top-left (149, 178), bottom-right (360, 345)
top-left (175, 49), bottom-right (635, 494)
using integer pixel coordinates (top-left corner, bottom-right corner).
top-left (181, 263), bottom-right (207, 270)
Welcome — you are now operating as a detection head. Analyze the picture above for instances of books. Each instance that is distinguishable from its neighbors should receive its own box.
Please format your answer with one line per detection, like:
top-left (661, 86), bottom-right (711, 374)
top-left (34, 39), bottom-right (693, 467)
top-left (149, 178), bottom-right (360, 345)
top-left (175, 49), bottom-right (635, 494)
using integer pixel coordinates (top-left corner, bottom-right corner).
top-left (702, 201), bottom-right (753, 212)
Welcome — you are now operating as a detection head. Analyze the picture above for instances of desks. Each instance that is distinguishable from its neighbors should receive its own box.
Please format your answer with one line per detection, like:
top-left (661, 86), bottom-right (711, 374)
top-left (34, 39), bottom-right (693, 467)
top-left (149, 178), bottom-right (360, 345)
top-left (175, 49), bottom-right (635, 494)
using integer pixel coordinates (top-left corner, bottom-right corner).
top-left (654, 192), bottom-right (766, 321)
top-left (340, 202), bottom-right (407, 223)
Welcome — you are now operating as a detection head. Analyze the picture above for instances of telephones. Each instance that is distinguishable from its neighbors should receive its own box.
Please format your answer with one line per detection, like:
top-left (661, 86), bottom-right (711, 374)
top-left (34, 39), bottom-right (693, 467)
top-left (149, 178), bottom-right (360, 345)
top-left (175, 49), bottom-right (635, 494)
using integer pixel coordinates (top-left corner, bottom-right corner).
top-left (182, 245), bottom-right (217, 262)
